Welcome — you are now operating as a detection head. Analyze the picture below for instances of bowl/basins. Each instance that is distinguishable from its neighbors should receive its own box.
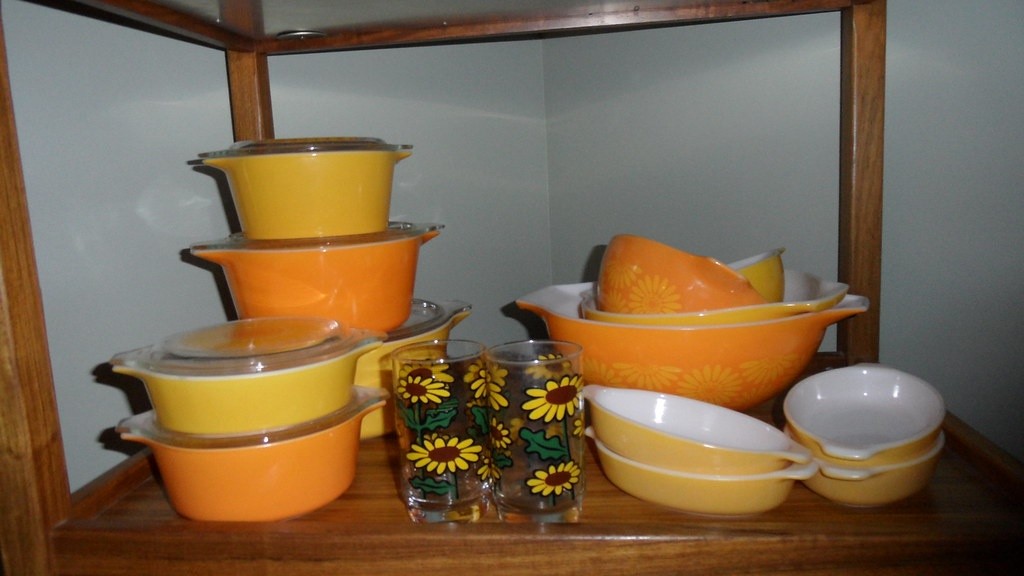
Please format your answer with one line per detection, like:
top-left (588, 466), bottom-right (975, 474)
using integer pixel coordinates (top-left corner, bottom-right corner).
top-left (784, 363), bottom-right (946, 510)
top-left (515, 234), bottom-right (870, 413)
top-left (110, 138), bottom-right (471, 522)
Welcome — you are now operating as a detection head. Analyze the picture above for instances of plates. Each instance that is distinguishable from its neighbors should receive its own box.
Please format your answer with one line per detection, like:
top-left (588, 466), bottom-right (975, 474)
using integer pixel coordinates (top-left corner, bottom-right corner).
top-left (583, 385), bottom-right (818, 516)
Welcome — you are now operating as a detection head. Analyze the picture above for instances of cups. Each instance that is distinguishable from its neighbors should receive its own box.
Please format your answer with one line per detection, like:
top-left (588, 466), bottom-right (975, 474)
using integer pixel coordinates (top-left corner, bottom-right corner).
top-left (390, 338), bottom-right (584, 527)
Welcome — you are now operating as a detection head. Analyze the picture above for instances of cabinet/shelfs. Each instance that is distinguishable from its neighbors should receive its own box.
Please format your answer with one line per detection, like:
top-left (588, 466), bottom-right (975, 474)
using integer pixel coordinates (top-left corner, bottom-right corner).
top-left (0, 0), bottom-right (1024, 576)
